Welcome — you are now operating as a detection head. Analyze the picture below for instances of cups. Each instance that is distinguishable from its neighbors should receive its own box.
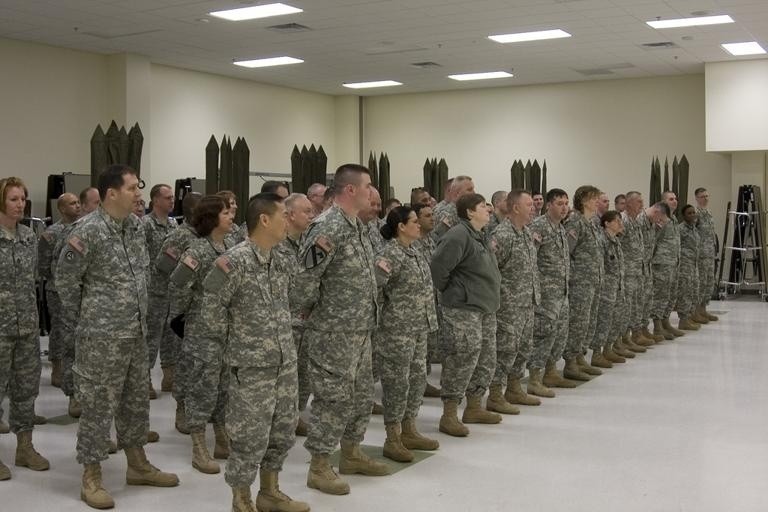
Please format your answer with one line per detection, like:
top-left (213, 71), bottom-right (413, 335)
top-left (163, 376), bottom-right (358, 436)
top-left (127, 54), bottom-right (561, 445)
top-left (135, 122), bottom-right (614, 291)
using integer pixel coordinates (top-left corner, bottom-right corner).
top-left (137, 200), bottom-right (145, 206)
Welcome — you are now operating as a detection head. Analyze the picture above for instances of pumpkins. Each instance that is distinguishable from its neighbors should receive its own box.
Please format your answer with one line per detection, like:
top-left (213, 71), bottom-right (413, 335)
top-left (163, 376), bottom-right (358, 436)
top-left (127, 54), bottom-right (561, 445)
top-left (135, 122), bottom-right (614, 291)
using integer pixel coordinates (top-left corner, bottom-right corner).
top-left (718, 184), bottom-right (768, 302)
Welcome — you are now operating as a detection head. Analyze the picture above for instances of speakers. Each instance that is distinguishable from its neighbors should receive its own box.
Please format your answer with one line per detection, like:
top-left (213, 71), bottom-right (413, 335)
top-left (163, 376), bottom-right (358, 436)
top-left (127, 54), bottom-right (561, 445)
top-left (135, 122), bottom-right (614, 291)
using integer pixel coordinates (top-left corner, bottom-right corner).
top-left (69, 395), bottom-right (81, 417)
top-left (149, 430), bottom-right (159, 443)
top-left (692, 308), bottom-right (707, 323)
top-left (613, 343), bottom-right (635, 358)
top-left (339, 439), bottom-right (391, 476)
top-left (109, 438), bottom-right (117, 454)
top-left (622, 336), bottom-right (646, 352)
top-left (307, 455), bottom-right (351, 495)
top-left (701, 307), bottom-right (718, 323)
top-left (642, 327), bottom-right (664, 342)
top-left (296, 417), bottom-right (312, 436)
top-left (52, 359), bottom-right (62, 387)
top-left (576, 357), bottom-right (602, 375)
top-left (591, 353), bottom-right (613, 368)
top-left (382, 438), bottom-right (414, 461)
top-left (424, 384), bottom-right (440, 397)
top-left (462, 397), bottom-right (502, 423)
top-left (191, 433), bottom-right (221, 474)
top-left (232, 486), bottom-right (258, 512)
top-left (506, 378), bottom-right (541, 406)
top-left (81, 464), bottom-right (115, 509)
top-left (564, 360), bottom-right (591, 381)
top-left (487, 385), bottom-right (519, 415)
top-left (662, 318), bottom-right (684, 337)
top-left (400, 418), bottom-right (439, 450)
top-left (602, 348), bottom-right (625, 363)
top-left (161, 366), bottom-right (174, 392)
top-left (678, 320), bottom-right (698, 330)
top-left (543, 370), bottom-right (577, 388)
top-left (15, 431), bottom-right (50, 471)
top-left (256, 469), bottom-right (310, 512)
top-left (0, 408), bottom-right (9, 433)
top-left (214, 435), bottom-right (231, 459)
top-left (439, 400), bottom-right (469, 435)
top-left (631, 331), bottom-right (655, 346)
top-left (653, 319), bottom-right (674, 340)
top-left (527, 369), bottom-right (555, 397)
top-left (176, 402), bottom-right (191, 435)
top-left (126, 444), bottom-right (179, 487)
top-left (0, 459), bottom-right (11, 480)
top-left (33, 416), bottom-right (46, 424)
top-left (372, 404), bottom-right (385, 414)
top-left (149, 381), bottom-right (157, 399)
top-left (687, 317), bottom-right (701, 328)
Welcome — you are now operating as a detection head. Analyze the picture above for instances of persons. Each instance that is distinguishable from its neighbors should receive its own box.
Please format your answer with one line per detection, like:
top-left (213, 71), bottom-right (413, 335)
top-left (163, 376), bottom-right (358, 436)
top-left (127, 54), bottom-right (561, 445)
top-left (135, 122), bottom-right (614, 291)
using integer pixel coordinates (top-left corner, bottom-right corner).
top-left (486, 190), bottom-right (541, 415)
top-left (0, 177), bottom-right (50, 481)
top-left (67, 188), bottom-right (100, 418)
top-left (631, 202), bottom-right (670, 347)
top-left (480, 190), bottom-right (509, 238)
top-left (678, 205), bottom-right (702, 331)
top-left (154, 191), bottom-right (215, 434)
top-left (612, 191), bottom-right (646, 359)
top-left (38, 192), bottom-right (82, 390)
top-left (382, 198), bottom-right (402, 222)
top-left (433, 178), bottom-right (455, 222)
top-left (563, 185), bottom-right (605, 381)
top-left (357, 186), bottom-right (383, 418)
top-left (413, 204), bottom-right (442, 404)
top-left (592, 210), bottom-right (626, 368)
top-left (199, 193), bottom-right (311, 512)
top-left (431, 176), bottom-right (476, 244)
top-left (216, 190), bottom-right (241, 241)
top-left (288, 165), bottom-right (391, 496)
top-left (168, 195), bottom-right (240, 474)
top-left (411, 187), bottom-right (431, 209)
top-left (614, 194), bottom-right (627, 212)
top-left (0, 381), bottom-right (49, 436)
top-left (693, 187), bottom-right (719, 324)
top-left (283, 192), bottom-right (314, 438)
top-left (597, 191), bottom-right (610, 218)
top-left (485, 202), bottom-right (494, 219)
top-left (530, 192), bottom-right (545, 221)
top-left (52, 209), bottom-right (160, 456)
top-left (373, 207), bottom-right (440, 462)
top-left (322, 187), bottom-right (339, 212)
top-left (650, 191), bottom-right (685, 340)
top-left (429, 193), bottom-right (503, 437)
top-left (139, 184), bottom-right (179, 399)
top-left (133, 192), bottom-right (146, 220)
top-left (526, 188), bottom-right (577, 398)
top-left (261, 179), bottom-right (289, 199)
top-left (306, 183), bottom-right (326, 217)
